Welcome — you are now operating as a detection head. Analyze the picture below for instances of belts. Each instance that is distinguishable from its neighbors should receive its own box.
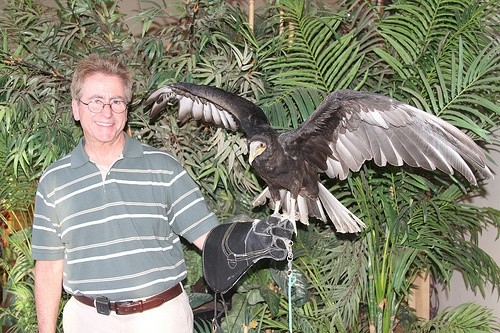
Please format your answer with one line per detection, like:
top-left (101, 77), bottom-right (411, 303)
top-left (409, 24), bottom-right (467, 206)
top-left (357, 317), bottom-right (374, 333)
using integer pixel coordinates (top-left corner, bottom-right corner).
top-left (73, 283), bottom-right (182, 316)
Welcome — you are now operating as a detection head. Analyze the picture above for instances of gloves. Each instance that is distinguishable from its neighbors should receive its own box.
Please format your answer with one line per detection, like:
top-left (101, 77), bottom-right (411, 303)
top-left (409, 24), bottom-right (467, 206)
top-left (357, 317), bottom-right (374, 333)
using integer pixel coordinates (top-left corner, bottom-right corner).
top-left (201, 216), bottom-right (293, 294)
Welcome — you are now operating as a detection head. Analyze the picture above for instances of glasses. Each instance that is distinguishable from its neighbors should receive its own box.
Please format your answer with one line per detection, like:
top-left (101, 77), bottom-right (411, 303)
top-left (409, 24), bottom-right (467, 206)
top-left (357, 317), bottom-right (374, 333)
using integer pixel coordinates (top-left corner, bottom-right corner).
top-left (78, 99), bottom-right (128, 114)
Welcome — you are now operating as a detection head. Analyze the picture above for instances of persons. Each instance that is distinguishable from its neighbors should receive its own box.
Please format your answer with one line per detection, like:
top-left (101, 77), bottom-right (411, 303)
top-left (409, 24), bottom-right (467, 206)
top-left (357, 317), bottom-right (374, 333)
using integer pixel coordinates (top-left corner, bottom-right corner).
top-left (29, 53), bottom-right (294, 333)
